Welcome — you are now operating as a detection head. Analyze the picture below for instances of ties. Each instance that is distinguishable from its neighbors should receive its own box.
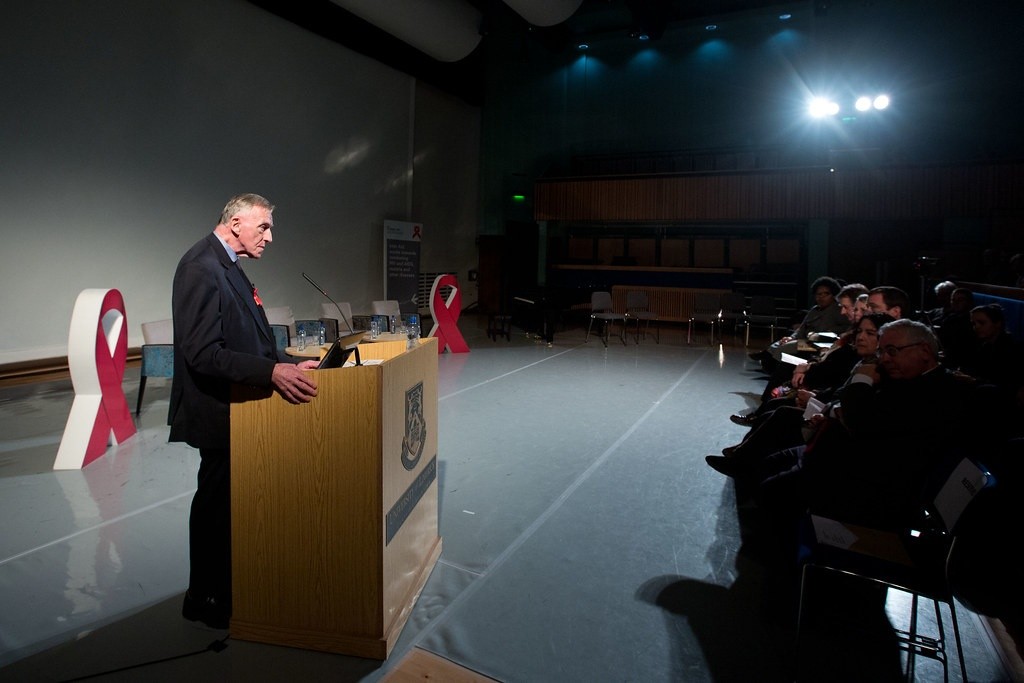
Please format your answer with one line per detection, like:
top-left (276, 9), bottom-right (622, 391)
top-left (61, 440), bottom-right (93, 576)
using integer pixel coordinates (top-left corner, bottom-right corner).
top-left (235, 259), bottom-right (242, 271)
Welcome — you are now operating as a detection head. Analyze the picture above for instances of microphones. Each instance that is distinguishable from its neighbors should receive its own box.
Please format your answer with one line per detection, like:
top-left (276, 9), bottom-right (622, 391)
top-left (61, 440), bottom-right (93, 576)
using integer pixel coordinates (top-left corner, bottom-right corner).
top-left (302, 272), bottom-right (364, 367)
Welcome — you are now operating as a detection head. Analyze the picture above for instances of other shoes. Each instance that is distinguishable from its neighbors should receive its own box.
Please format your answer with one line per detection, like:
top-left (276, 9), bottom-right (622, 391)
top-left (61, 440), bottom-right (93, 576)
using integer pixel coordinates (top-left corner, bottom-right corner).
top-left (730, 412), bottom-right (762, 428)
top-left (705, 455), bottom-right (740, 481)
top-left (748, 353), bottom-right (760, 360)
top-left (181, 588), bottom-right (230, 630)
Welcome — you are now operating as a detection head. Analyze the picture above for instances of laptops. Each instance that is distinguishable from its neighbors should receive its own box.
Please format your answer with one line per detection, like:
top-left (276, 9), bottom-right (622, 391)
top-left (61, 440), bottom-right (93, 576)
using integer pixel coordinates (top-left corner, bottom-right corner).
top-left (316, 331), bottom-right (368, 369)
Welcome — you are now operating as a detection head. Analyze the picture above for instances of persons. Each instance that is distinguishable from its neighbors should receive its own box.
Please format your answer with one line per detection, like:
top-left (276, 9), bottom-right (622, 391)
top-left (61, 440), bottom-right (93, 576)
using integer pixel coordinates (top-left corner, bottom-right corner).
top-left (167, 193), bottom-right (318, 629)
top-left (706, 275), bottom-right (1024, 657)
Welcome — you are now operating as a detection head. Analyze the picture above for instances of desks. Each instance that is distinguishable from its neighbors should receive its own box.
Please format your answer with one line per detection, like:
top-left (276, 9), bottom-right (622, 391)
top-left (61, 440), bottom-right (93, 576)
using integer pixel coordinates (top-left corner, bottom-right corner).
top-left (284, 343), bottom-right (334, 361)
top-left (362, 331), bottom-right (407, 342)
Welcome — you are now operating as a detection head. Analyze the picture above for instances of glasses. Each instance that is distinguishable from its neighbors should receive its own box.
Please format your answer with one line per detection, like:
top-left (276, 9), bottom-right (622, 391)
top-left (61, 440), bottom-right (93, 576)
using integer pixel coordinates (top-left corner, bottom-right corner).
top-left (875, 342), bottom-right (925, 359)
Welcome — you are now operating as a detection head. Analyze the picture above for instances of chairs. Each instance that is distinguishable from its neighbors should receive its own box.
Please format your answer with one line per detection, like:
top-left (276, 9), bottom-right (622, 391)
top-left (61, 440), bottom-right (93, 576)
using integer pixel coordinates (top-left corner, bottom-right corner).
top-left (579, 285), bottom-right (1023, 682)
top-left (135, 301), bottom-right (421, 415)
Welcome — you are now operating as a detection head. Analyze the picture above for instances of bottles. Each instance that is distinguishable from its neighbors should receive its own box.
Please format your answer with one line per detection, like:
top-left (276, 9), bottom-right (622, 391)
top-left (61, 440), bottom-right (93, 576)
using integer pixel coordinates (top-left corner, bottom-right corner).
top-left (390, 315), bottom-right (396, 333)
top-left (318, 323), bottom-right (325, 345)
top-left (298, 325), bottom-right (306, 351)
top-left (407, 316), bottom-right (421, 349)
top-left (370, 314), bottom-right (387, 337)
